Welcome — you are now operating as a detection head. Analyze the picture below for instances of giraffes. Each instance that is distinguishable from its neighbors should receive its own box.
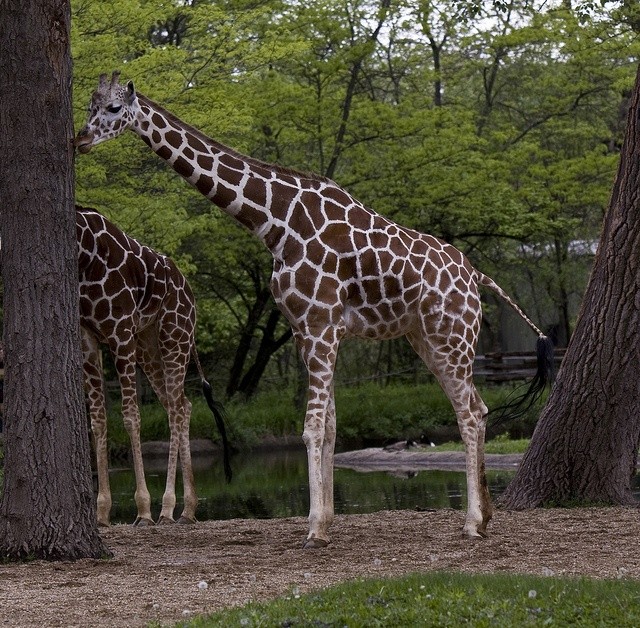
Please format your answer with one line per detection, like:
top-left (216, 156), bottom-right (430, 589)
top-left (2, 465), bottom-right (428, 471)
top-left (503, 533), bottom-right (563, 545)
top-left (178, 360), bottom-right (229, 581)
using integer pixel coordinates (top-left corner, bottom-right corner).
top-left (75, 204), bottom-right (239, 528)
top-left (73, 70), bottom-right (554, 548)
